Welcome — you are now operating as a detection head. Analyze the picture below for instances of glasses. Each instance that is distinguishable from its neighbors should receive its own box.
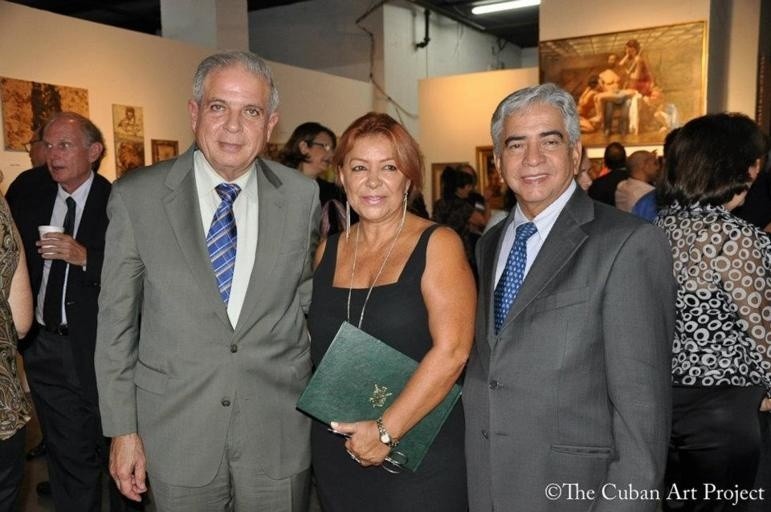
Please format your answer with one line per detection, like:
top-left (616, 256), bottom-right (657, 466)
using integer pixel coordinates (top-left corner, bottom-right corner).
top-left (311, 140), bottom-right (332, 153)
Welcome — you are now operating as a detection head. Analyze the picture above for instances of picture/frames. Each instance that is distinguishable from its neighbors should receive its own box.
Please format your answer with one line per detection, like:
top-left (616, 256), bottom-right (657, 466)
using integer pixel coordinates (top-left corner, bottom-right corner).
top-left (151, 137), bottom-right (177, 162)
top-left (429, 162), bottom-right (467, 207)
top-left (538, 21), bottom-right (705, 150)
top-left (476, 146), bottom-right (504, 208)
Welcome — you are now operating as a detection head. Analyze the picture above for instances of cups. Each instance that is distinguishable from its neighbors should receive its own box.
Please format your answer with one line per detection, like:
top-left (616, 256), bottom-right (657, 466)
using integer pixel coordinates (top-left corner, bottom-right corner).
top-left (38, 225), bottom-right (67, 260)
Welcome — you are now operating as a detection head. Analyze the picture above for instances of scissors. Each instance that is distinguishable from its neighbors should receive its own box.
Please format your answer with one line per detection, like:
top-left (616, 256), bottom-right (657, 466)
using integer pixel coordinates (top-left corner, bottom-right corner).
top-left (327, 428), bottom-right (408, 474)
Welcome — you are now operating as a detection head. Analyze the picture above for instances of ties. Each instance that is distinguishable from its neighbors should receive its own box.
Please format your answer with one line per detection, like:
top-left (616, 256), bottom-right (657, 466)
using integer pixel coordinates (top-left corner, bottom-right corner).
top-left (41, 198), bottom-right (75, 332)
top-left (207, 183), bottom-right (241, 308)
top-left (493, 222), bottom-right (538, 337)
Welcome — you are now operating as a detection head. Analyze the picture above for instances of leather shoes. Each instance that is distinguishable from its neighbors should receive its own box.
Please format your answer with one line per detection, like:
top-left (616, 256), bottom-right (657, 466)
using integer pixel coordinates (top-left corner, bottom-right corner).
top-left (37, 481), bottom-right (51, 495)
top-left (29, 439), bottom-right (48, 458)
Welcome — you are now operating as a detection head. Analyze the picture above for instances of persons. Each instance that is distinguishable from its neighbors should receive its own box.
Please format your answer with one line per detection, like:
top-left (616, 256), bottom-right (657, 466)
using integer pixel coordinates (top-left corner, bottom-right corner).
top-left (619, 38), bottom-right (649, 92)
